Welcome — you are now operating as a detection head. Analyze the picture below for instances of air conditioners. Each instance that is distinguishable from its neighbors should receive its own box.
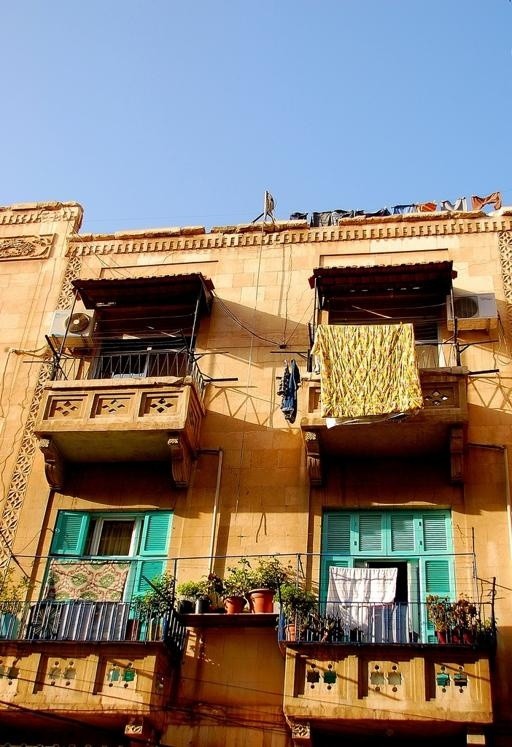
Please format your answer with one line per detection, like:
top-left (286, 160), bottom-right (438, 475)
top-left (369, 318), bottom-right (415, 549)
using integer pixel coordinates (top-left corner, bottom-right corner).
top-left (48, 310), bottom-right (95, 347)
top-left (447, 293), bottom-right (498, 331)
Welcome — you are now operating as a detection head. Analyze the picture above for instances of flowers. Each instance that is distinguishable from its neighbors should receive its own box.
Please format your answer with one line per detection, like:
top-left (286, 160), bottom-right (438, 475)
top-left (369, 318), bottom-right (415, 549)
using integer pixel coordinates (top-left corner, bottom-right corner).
top-left (426, 595), bottom-right (452, 631)
top-left (452, 595), bottom-right (478, 630)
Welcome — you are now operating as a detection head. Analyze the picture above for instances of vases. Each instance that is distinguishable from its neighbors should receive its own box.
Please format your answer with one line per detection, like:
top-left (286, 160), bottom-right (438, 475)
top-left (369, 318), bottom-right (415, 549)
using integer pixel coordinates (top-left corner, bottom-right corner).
top-left (436, 629), bottom-right (446, 644)
top-left (455, 624), bottom-right (472, 644)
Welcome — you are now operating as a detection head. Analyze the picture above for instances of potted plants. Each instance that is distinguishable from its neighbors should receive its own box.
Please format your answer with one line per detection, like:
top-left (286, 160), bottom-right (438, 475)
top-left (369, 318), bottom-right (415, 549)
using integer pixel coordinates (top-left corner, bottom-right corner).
top-left (196, 575), bottom-right (210, 613)
top-left (273, 585), bottom-right (317, 641)
top-left (136, 573), bottom-right (173, 640)
top-left (247, 559), bottom-right (282, 613)
top-left (177, 581), bottom-right (196, 613)
top-left (0, 566), bottom-right (32, 639)
top-left (223, 568), bottom-right (246, 613)
